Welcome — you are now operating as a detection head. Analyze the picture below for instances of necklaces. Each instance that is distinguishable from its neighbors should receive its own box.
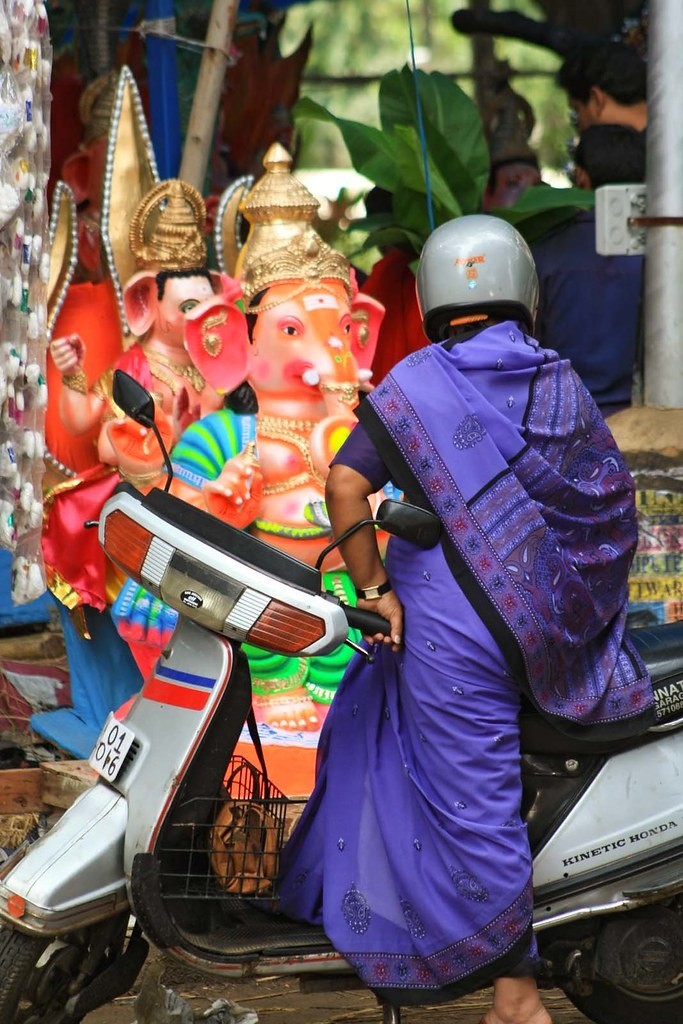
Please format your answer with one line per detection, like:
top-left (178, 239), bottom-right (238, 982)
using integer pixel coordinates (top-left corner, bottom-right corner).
top-left (141, 341), bottom-right (207, 394)
top-left (254, 417), bottom-right (317, 435)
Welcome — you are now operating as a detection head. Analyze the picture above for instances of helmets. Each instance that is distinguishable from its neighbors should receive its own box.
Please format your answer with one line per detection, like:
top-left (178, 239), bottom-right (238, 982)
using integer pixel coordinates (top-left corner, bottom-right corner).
top-left (410, 213), bottom-right (543, 341)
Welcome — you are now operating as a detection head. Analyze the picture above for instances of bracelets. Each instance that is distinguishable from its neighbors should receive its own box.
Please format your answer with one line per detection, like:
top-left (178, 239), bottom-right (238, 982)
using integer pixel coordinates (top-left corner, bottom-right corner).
top-left (60, 373), bottom-right (89, 394)
top-left (355, 578), bottom-right (393, 599)
top-left (118, 465), bottom-right (162, 489)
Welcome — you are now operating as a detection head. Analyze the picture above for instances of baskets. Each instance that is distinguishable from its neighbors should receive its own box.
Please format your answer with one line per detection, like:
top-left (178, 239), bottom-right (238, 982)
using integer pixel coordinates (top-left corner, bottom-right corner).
top-left (157, 754), bottom-right (290, 900)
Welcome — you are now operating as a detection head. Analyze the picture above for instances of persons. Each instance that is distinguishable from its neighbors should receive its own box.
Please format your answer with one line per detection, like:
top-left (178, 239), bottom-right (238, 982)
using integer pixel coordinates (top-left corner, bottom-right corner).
top-left (472, 147), bottom-right (542, 215)
top-left (272, 210), bottom-right (657, 1024)
top-left (553, 36), bottom-right (648, 140)
top-left (33, 177), bottom-right (406, 734)
top-left (529, 123), bottom-right (645, 419)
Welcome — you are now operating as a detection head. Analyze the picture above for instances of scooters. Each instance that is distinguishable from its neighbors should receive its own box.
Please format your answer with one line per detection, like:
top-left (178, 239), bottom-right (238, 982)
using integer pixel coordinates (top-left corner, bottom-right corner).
top-left (0, 369), bottom-right (683, 1024)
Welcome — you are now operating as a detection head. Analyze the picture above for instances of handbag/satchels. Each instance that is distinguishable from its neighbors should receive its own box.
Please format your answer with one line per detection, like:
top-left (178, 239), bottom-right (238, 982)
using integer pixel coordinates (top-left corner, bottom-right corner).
top-left (207, 799), bottom-right (278, 894)
